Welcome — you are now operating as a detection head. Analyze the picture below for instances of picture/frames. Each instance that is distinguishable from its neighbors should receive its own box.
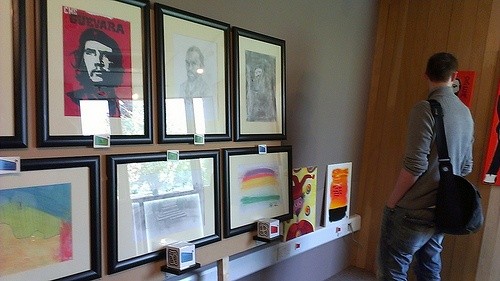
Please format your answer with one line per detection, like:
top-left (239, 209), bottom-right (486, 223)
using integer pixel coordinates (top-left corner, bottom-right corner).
top-left (0, 155), bottom-right (102, 281)
top-left (106, 149), bottom-right (222, 275)
top-left (36, 0), bottom-right (153, 144)
top-left (0, 0), bottom-right (29, 149)
top-left (232, 26), bottom-right (287, 142)
top-left (222, 145), bottom-right (294, 239)
top-left (152, 2), bottom-right (233, 143)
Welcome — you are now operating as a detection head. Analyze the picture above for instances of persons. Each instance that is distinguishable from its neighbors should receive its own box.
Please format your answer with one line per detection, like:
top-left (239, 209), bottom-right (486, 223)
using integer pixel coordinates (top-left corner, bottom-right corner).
top-left (376, 52), bottom-right (475, 281)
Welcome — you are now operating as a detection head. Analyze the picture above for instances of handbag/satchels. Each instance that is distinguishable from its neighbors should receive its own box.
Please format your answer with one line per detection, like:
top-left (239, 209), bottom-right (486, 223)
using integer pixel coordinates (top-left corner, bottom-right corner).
top-left (432, 161), bottom-right (485, 236)
